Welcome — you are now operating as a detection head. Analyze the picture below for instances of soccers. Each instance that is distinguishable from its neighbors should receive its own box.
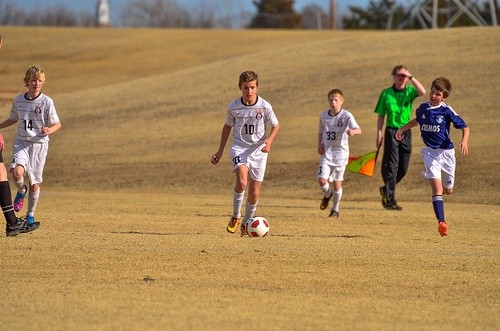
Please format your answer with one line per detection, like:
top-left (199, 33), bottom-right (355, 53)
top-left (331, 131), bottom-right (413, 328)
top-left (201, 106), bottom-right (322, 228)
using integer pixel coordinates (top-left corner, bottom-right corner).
top-left (245, 216), bottom-right (269, 237)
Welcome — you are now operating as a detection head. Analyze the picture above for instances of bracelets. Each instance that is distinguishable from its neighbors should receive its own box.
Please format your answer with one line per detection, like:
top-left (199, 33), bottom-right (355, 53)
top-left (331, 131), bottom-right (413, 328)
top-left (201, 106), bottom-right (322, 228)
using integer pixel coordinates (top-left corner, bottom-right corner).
top-left (408, 75), bottom-right (414, 80)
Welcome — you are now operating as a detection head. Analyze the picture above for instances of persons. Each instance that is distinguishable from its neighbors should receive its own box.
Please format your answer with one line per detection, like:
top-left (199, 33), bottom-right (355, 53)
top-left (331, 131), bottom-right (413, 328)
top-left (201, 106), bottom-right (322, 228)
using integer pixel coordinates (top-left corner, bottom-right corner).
top-left (395, 77), bottom-right (470, 237)
top-left (0, 32), bottom-right (40, 238)
top-left (210, 71), bottom-right (280, 235)
top-left (0, 64), bottom-right (62, 224)
top-left (373, 65), bottom-right (426, 210)
top-left (316, 89), bottom-right (362, 218)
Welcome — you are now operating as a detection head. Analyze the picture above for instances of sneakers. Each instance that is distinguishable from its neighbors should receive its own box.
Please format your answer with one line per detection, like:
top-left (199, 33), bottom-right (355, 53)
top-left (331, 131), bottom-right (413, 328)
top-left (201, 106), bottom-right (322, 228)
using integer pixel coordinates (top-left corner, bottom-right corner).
top-left (438, 221), bottom-right (448, 237)
top-left (320, 189), bottom-right (333, 210)
top-left (240, 223), bottom-right (249, 237)
top-left (329, 209), bottom-right (339, 218)
top-left (227, 216), bottom-right (240, 233)
top-left (14, 184), bottom-right (27, 212)
top-left (380, 186), bottom-right (388, 208)
top-left (26, 215), bottom-right (34, 224)
top-left (385, 200), bottom-right (402, 210)
top-left (6, 218), bottom-right (40, 237)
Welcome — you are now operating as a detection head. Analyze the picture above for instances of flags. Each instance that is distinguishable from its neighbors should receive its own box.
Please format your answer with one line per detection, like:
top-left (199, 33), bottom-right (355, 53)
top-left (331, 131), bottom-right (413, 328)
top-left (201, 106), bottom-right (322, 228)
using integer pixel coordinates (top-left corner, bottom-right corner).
top-left (347, 150), bottom-right (379, 177)
